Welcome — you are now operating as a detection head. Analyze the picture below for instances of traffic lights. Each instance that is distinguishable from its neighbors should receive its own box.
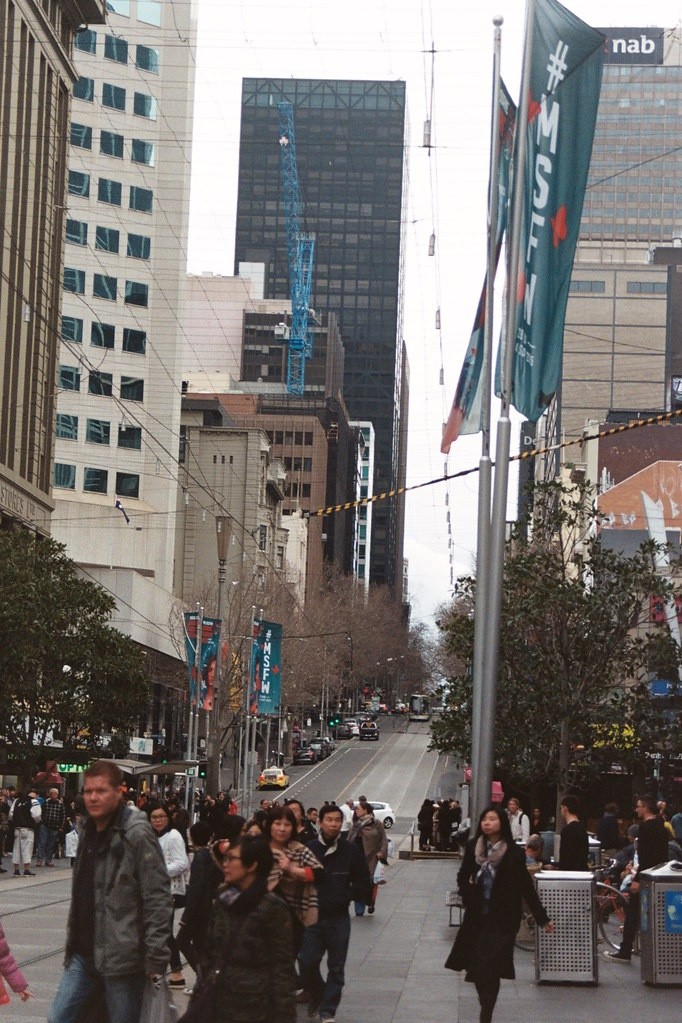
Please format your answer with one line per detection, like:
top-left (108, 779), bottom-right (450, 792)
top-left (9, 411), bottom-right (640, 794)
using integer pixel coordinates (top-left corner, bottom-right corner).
top-left (327, 715), bottom-right (340, 727)
top-left (198, 760), bottom-right (207, 779)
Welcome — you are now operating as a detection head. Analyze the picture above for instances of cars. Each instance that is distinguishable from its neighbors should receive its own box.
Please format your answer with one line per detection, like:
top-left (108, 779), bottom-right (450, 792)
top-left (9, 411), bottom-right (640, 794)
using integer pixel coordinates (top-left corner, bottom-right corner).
top-left (354, 801), bottom-right (396, 829)
top-left (259, 766), bottom-right (290, 792)
top-left (332, 694), bottom-right (434, 741)
top-left (293, 737), bottom-right (334, 766)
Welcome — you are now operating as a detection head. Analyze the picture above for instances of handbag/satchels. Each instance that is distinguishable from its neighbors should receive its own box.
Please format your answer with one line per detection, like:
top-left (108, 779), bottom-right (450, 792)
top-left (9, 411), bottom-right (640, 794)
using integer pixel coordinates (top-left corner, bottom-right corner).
top-left (373, 859), bottom-right (390, 885)
top-left (143, 977), bottom-right (180, 1023)
top-left (65, 830), bottom-right (80, 858)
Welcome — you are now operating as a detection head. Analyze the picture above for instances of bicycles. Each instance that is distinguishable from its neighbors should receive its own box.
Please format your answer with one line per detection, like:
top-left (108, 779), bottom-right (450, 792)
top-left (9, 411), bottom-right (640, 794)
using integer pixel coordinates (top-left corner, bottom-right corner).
top-left (513, 857), bottom-right (641, 955)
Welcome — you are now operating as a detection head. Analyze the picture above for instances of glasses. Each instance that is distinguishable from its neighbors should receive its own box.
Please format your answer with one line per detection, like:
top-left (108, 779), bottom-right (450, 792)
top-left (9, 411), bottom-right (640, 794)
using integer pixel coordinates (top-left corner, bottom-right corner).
top-left (150, 814), bottom-right (167, 820)
top-left (223, 855), bottom-right (243, 862)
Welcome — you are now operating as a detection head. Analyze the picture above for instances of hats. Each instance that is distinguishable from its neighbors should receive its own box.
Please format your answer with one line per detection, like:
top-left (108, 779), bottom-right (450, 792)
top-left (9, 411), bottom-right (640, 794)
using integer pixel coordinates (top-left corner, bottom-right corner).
top-left (214, 815), bottom-right (247, 840)
top-left (627, 824), bottom-right (639, 835)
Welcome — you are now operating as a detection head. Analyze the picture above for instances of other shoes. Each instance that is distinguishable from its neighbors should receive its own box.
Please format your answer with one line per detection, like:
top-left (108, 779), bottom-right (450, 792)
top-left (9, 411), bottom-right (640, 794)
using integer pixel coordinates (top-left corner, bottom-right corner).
top-left (36, 864), bottom-right (44, 867)
top-left (45, 863), bottom-right (54, 867)
top-left (184, 988), bottom-right (194, 995)
top-left (604, 949), bottom-right (632, 962)
top-left (368, 905), bottom-right (376, 914)
top-left (0, 867), bottom-right (8, 873)
top-left (23, 870), bottom-right (36, 877)
top-left (165, 979), bottom-right (187, 989)
top-left (356, 912), bottom-right (364, 918)
top-left (297, 991), bottom-right (308, 1004)
top-left (306, 1007), bottom-right (319, 1021)
top-left (13, 871), bottom-right (21, 877)
top-left (320, 1011), bottom-right (336, 1023)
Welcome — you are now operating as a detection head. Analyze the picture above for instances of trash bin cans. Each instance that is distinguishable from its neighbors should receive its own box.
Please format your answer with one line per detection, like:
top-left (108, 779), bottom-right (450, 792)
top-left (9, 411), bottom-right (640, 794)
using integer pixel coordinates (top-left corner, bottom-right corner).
top-left (275, 753), bottom-right (284, 766)
top-left (534, 870), bottom-right (599, 986)
top-left (638, 859), bottom-right (682, 988)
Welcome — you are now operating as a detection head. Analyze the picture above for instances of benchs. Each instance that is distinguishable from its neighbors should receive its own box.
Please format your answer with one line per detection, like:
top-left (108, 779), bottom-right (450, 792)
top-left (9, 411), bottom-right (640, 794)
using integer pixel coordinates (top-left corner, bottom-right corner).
top-left (446, 888), bottom-right (464, 927)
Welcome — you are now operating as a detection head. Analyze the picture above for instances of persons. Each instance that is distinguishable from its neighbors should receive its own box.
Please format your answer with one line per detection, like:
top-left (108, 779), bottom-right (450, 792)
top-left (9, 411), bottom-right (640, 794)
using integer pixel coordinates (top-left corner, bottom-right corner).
top-left (0, 783), bottom-right (87, 878)
top-left (0, 923), bottom-right (36, 1002)
top-left (557, 796), bottom-right (589, 871)
top-left (201, 655), bottom-right (217, 711)
top-left (418, 798), bottom-right (462, 852)
top-left (122, 780), bottom-right (388, 1023)
top-left (597, 794), bottom-right (682, 963)
top-left (444, 805), bottom-right (554, 1023)
top-left (47, 762), bottom-right (175, 1023)
top-left (505, 798), bottom-right (545, 863)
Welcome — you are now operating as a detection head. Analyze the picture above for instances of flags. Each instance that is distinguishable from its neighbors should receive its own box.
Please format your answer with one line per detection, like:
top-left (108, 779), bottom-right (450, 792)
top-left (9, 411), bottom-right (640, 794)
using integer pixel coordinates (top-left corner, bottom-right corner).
top-left (441, 0), bottom-right (607, 454)
